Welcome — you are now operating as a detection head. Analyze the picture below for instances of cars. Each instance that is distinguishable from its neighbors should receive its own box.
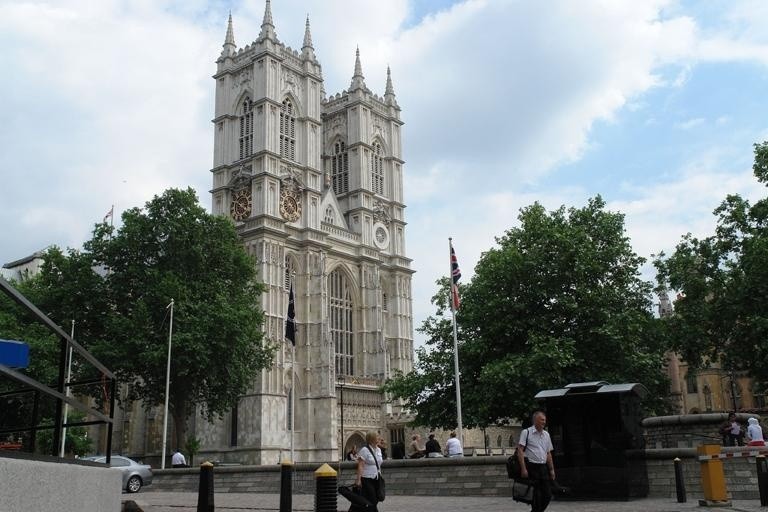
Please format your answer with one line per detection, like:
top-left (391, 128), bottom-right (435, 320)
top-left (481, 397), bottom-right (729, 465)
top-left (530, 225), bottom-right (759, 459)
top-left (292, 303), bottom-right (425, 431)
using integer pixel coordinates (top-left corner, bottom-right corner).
top-left (77, 453), bottom-right (154, 495)
top-left (0, 441), bottom-right (22, 452)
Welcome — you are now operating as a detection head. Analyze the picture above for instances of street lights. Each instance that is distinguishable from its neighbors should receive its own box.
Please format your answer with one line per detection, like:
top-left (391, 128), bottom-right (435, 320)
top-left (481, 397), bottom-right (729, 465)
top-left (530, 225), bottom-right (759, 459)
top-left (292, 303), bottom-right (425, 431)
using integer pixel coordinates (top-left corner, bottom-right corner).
top-left (336, 373), bottom-right (345, 462)
top-left (727, 369), bottom-right (737, 414)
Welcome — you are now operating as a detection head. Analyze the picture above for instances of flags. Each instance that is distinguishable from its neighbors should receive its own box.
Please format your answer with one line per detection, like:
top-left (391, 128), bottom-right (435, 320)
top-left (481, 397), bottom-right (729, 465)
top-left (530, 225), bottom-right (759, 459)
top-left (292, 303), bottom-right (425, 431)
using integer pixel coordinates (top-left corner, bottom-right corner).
top-left (450, 244), bottom-right (462, 308)
top-left (285, 279), bottom-right (297, 345)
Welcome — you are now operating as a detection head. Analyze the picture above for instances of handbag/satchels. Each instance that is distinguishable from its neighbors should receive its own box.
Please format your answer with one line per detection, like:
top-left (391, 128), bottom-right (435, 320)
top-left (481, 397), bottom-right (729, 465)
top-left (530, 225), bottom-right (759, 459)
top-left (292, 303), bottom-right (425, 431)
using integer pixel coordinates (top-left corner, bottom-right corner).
top-left (511, 481), bottom-right (535, 502)
top-left (375, 472), bottom-right (386, 503)
top-left (554, 479), bottom-right (572, 495)
top-left (338, 483), bottom-right (374, 510)
top-left (506, 427), bottom-right (530, 480)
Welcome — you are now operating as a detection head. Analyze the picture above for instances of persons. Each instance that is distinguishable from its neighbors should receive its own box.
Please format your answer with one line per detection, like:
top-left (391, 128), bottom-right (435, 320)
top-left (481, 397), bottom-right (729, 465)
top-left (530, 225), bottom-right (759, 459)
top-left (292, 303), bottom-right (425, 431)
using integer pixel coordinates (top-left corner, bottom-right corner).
top-left (347, 445), bottom-right (358, 461)
top-left (424, 434), bottom-right (442, 458)
top-left (442, 431), bottom-right (464, 458)
top-left (347, 431), bottom-right (385, 511)
top-left (376, 440), bottom-right (390, 460)
top-left (743, 416), bottom-right (766, 447)
top-left (516, 411), bottom-right (557, 511)
top-left (172, 447), bottom-right (188, 467)
top-left (719, 413), bottom-right (747, 450)
top-left (406, 433), bottom-right (427, 459)
